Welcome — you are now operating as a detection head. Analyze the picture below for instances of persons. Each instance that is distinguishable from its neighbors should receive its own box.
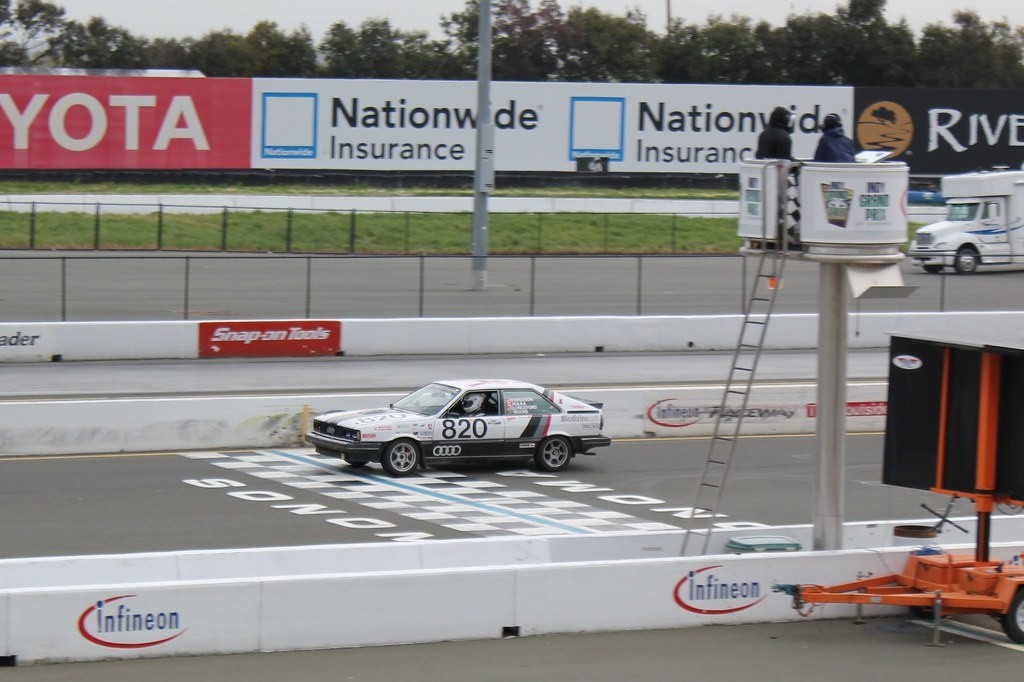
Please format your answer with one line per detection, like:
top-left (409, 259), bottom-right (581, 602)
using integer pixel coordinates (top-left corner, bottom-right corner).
top-left (813, 113), bottom-right (856, 164)
top-left (755, 106), bottom-right (801, 250)
top-left (461, 392), bottom-right (486, 417)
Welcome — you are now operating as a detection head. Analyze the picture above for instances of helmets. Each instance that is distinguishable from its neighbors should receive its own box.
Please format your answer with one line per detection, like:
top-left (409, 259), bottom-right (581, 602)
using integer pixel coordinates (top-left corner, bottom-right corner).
top-left (463, 393), bottom-right (484, 413)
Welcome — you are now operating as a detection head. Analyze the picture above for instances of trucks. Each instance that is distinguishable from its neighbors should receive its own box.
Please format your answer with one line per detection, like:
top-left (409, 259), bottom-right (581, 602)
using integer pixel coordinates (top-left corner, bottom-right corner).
top-left (905, 170), bottom-right (1023, 275)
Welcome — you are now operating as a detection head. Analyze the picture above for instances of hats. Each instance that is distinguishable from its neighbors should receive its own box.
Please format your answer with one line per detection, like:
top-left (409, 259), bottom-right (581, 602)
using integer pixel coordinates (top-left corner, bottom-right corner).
top-left (825, 114), bottom-right (840, 126)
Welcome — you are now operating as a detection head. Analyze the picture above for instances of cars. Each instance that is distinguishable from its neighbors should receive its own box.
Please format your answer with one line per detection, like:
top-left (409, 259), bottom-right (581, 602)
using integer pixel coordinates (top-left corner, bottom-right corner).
top-left (305, 381), bottom-right (612, 476)
